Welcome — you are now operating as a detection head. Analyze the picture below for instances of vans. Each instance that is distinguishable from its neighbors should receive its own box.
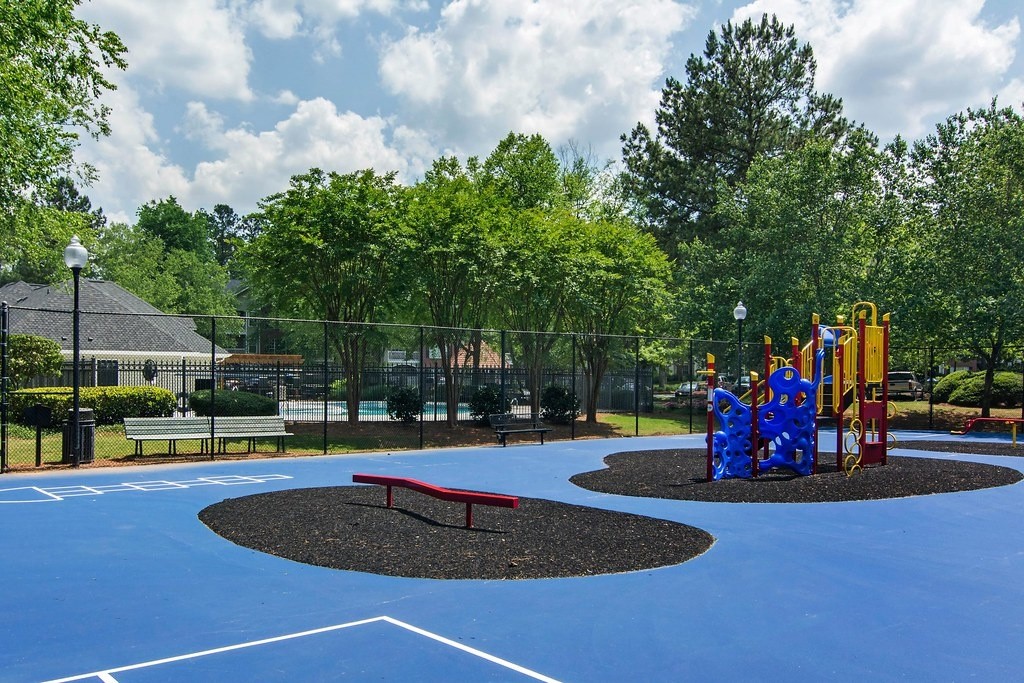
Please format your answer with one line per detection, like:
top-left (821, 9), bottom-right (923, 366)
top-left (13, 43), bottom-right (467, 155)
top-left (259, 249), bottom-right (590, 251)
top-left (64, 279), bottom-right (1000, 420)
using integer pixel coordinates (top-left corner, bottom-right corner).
top-left (717, 375), bottom-right (729, 387)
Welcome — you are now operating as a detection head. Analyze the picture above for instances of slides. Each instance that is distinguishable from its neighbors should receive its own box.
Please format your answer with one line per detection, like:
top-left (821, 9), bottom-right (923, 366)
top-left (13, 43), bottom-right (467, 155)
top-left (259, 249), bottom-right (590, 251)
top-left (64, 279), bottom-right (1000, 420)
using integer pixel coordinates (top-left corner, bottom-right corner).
top-left (723, 340), bottom-right (812, 429)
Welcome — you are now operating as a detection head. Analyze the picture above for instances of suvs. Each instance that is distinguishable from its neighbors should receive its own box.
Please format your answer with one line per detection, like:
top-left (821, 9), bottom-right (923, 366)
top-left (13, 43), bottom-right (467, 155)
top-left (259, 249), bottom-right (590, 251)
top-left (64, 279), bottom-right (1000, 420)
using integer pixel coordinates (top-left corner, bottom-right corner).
top-left (887, 370), bottom-right (924, 400)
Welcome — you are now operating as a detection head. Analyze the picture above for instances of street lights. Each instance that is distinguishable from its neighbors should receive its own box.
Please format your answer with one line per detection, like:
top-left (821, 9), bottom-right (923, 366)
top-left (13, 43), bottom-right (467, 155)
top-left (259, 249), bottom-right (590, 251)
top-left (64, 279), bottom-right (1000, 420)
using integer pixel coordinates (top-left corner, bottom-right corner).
top-left (64, 233), bottom-right (90, 465)
top-left (733, 300), bottom-right (748, 399)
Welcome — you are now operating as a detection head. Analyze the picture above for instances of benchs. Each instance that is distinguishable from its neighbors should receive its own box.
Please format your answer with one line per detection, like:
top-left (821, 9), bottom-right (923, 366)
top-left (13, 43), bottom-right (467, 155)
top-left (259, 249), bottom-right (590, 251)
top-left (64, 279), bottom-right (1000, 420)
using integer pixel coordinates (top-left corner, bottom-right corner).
top-left (123, 417), bottom-right (211, 458)
top-left (208, 416), bottom-right (295, 455)
top-left (488, 413), bottom-right (554, 447)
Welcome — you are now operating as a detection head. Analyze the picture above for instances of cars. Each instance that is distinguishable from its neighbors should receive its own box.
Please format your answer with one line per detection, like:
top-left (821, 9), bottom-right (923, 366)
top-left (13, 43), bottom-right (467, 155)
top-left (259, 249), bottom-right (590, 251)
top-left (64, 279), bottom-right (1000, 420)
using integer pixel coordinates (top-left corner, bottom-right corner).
top-left (675, 382), bottom-right (700, 397)
top-left (693, 382), bottom-right (708, 397)
top-left (734, 375), bottom-right (752, 389)
